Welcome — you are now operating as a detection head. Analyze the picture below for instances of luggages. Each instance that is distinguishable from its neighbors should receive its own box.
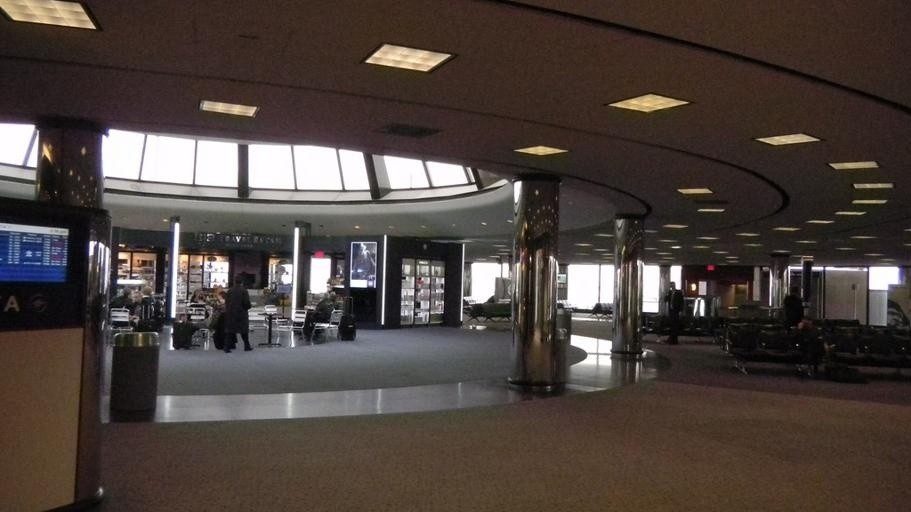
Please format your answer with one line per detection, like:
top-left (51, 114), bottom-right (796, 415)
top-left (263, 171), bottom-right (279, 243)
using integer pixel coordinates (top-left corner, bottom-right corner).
top-left (173, 302), bottom-right (193, 349)
top-left (340, 297), bottom-right (355, 340)
top-left (138, 300), bottom-right (152, 331)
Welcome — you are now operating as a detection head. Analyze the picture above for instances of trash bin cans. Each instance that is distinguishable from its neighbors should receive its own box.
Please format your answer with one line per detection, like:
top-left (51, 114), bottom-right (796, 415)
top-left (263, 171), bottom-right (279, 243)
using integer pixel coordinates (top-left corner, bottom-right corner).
top-left (553, 308), bottom-right (572, 383)
top-left (110, 332), bottom-right (159, 411)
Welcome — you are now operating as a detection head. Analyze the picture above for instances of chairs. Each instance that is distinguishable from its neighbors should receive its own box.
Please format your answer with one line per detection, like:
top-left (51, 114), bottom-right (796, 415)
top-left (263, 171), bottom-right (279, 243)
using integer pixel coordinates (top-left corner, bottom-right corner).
top-left (107, 308), bottom-right (134, 346)
top-left (264, 304), bottom-right (344, 344)
top-left (655, 315), bottom-right (911, 380)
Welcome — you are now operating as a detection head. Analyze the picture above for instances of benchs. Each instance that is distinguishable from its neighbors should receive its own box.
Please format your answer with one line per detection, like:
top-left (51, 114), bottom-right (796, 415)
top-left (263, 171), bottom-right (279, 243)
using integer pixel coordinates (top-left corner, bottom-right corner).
top-left (589, 303), bottom-right (614, 319)
top-left (463, 303), bottom-right (512, 323)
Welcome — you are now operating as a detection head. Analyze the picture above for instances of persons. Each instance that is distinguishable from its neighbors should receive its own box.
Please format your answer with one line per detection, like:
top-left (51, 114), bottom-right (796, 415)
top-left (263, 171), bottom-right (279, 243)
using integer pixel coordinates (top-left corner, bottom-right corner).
top-left (662, 280), bottom-right (830, 379)
top-left (107, 273), bottom-right (344, 352)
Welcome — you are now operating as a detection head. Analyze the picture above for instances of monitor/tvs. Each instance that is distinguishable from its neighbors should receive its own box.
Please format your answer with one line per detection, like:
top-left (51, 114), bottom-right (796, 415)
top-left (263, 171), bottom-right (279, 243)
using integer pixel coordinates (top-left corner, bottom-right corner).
top-left (2, 219), bottom-right (67, 286)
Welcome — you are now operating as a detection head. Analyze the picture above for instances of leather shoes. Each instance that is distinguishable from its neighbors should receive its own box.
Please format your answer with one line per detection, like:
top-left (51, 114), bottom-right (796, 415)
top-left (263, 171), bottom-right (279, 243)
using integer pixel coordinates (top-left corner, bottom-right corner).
top-left (244, 347), bottom-right (253, 351)
top-left (223, 347), bottom-right (231, 352)
